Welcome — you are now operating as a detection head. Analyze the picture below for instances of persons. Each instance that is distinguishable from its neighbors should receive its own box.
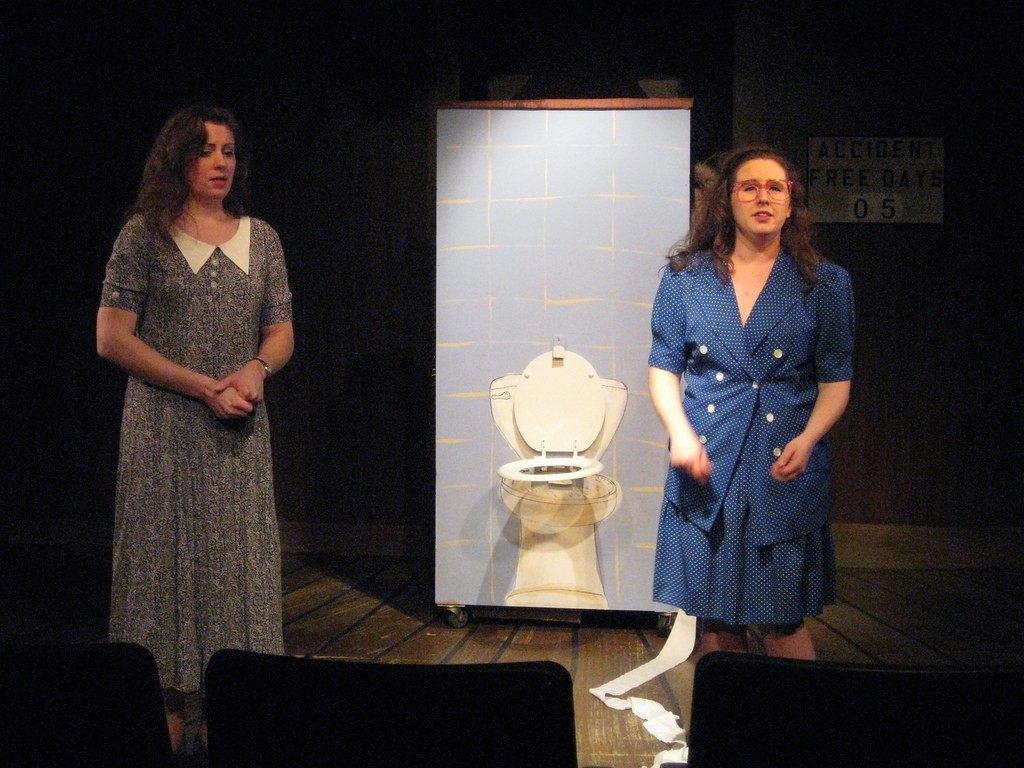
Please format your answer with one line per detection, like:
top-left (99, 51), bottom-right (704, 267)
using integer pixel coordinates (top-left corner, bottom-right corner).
top-left (93, 109), bottom-right (297, 760)
top-left (649, 145), bottom-right (856, 658)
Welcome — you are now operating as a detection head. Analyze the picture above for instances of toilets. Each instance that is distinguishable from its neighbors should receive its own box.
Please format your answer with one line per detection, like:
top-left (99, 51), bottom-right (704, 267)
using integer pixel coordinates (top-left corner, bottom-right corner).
top-left (497, 345), bottom-right (606, 482)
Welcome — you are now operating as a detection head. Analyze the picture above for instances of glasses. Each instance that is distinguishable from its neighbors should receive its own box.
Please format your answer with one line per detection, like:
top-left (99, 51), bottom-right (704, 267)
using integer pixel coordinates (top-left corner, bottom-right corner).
top-left (730, 178), bottom-right (797, 202)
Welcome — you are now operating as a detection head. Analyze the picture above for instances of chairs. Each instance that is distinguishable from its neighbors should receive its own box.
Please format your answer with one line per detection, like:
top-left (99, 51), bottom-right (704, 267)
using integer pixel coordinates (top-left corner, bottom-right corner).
top-left (686, 651), bottom-right (1024, 768)
top-left (0, 643), bottom-right (174, 768)
top-left (204, 649), bottom-right (576, 768)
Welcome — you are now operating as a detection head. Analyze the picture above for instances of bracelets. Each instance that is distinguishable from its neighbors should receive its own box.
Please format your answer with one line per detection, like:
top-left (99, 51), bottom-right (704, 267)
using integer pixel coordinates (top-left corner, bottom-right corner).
top-left (250, 357), bottom-right (272, 377)
